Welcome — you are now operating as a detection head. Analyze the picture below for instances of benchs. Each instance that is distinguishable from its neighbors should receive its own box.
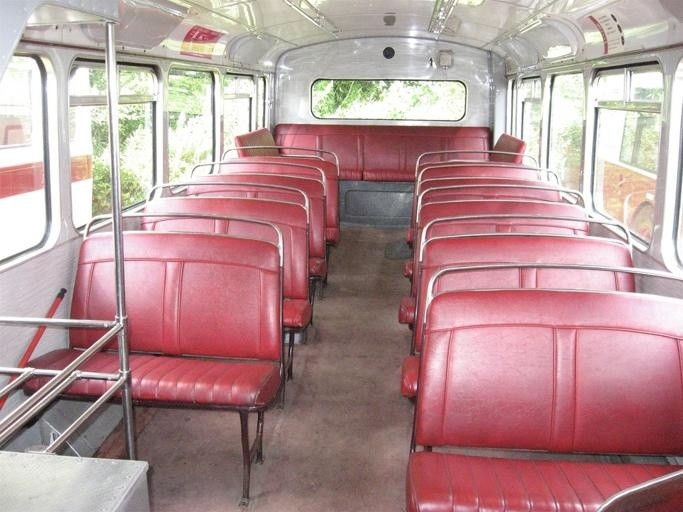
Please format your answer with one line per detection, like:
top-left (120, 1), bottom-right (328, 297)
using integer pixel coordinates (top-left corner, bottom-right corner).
top-left (18, 211), bottom-right (286, 505)
top-left (17, 145), bottom-right (338, 512)
top-left (228, 123), bottom-right (524, 184)
top-left (404, 261), bottom-right (682, 512)
top-left (186, 147), bottom-right (338, 304)
top-left (142, 181), bottom-right (312, 411)
top-left (597, 470), bottom-right (683, 512)
top-left (398, 153), bottom-right (683, 451)
